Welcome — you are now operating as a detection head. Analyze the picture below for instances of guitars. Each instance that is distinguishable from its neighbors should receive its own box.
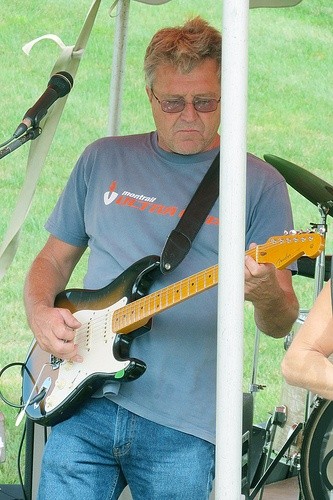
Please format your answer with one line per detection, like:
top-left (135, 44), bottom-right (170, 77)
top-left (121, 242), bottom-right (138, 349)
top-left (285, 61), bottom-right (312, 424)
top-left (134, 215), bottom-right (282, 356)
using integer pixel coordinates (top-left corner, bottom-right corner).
top-left (19, 221), bottom-right (330, 428)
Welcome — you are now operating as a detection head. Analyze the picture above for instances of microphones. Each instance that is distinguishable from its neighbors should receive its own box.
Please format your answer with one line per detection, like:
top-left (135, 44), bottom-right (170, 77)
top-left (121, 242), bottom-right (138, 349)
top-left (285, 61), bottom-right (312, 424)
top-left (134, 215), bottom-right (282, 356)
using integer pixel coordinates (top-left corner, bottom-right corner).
top-left (13, 71), bottom-right (74, 138)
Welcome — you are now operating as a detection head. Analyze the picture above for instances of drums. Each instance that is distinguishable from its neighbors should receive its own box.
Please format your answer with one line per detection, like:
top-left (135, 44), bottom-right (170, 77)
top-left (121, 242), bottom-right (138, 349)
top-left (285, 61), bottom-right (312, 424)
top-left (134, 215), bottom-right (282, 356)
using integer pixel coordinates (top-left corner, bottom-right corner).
top-left (293, 395), bottom-right (333, 500)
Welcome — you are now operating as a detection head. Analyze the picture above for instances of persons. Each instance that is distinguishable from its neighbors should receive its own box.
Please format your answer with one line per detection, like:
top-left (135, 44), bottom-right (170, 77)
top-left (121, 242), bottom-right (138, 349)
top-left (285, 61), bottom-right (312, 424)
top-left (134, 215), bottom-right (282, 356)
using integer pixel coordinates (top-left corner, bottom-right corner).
top-left (23, 15), bottom-right (300, 500)
top-left (280, 255), bottom-right (333, 404)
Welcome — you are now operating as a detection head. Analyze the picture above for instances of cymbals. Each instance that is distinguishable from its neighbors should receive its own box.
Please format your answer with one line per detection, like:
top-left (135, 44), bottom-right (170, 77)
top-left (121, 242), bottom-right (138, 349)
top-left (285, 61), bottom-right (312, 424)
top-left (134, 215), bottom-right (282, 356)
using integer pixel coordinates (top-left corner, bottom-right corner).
top-left (263, 152), bottom-right (332, 218)
top-left (294, 252), bottom-right (332, 283)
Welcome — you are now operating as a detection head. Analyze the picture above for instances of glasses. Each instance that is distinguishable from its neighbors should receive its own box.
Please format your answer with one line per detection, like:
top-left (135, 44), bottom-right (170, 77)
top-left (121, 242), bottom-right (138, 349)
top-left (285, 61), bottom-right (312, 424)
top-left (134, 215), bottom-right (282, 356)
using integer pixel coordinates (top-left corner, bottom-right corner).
top-left (150, 88), bottom-right (221, 113)
